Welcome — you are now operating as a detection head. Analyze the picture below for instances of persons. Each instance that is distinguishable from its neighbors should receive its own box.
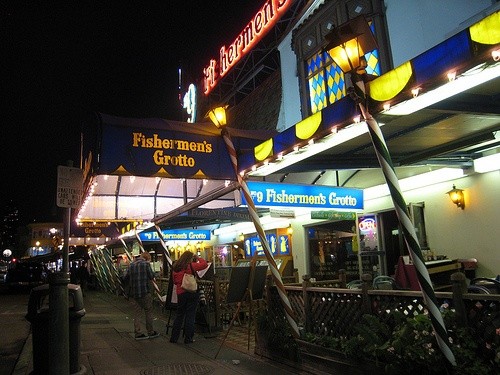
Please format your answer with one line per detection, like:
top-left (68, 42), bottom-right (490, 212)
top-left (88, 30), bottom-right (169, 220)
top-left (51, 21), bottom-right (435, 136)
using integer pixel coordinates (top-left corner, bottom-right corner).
top-left (170, 251), bottom-right (208, 344)
top-left (123, 252), bottom-right (160, 341)
top-left (70, 261), bottom-right (90, 292)
top-left (236, 254), bottom-right (244, 259)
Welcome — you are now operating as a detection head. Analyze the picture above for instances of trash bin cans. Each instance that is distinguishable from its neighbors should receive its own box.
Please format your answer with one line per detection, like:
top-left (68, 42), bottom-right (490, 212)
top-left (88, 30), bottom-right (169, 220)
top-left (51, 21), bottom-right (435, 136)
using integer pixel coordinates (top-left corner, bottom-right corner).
top-left (23, 282), bottom-right (86, 375)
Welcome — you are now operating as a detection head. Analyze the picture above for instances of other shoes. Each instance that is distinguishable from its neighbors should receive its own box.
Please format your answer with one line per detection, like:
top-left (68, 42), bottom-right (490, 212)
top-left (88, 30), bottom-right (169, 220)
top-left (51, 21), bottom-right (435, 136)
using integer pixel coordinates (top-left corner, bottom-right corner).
top-left (184, 339), bottom-right (194, 345)
top-left (170, 337), bottom-right (183, 343)
top-left (149, 331), bottom-right (160, 338)
top-left (135, 334), bottom-right (149, 340)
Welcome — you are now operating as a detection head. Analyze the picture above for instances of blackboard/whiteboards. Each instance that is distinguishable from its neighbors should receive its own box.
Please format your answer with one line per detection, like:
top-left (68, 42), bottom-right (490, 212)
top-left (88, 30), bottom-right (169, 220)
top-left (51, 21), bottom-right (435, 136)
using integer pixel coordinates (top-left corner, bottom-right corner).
top-left (164, 261), bottom-right (180, 311)
top-left (225, 266), bottom-right (268, 303)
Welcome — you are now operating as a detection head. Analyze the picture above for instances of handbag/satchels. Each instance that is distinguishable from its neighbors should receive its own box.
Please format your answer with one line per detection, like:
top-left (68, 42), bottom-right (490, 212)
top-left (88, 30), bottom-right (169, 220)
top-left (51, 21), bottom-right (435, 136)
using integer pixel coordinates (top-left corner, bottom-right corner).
top-left (181, 263), bottom-right (197, 291)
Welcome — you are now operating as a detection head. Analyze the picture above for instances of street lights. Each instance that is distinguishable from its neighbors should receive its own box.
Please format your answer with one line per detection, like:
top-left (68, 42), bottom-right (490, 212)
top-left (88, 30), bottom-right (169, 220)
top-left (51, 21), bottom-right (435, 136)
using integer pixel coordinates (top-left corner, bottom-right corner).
top-left (325, 28), bottom-right (457, 372)
top-left (204, 102), bottom-right (302, 340)
